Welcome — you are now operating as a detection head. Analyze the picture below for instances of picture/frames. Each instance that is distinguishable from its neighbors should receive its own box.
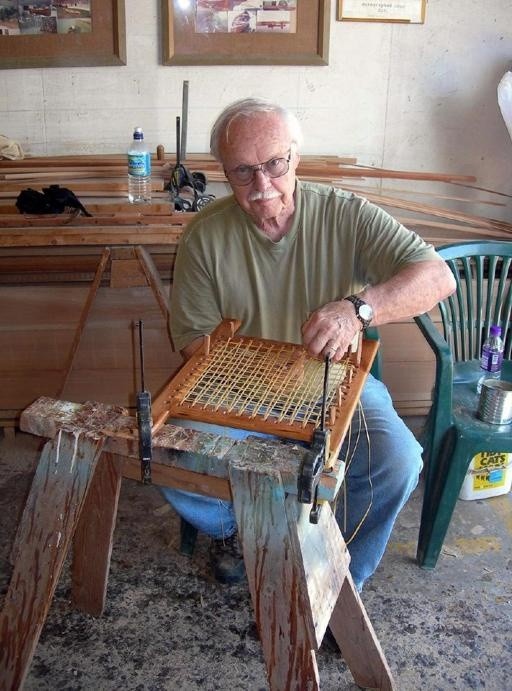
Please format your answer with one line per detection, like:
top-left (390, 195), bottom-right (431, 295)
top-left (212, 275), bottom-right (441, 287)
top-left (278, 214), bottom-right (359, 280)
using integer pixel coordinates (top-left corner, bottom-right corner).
top-left (0, 0), bottom-right (127, 70)
top-left (160, 0), bottom-right (331, 66)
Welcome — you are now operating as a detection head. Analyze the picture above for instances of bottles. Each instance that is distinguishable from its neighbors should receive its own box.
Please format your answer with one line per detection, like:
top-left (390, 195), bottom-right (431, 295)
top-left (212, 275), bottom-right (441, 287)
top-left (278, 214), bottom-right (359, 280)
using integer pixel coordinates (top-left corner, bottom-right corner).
top-left (127, 126), bottom-right (152, 203)
top-left (476, 326), bottom-right (505, 395)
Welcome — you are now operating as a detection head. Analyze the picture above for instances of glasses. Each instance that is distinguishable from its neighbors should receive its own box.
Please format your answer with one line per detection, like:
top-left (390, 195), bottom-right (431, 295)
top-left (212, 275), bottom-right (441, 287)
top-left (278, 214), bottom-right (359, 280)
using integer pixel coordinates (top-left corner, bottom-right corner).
top-left (223, 152), bottom-right (290, 186)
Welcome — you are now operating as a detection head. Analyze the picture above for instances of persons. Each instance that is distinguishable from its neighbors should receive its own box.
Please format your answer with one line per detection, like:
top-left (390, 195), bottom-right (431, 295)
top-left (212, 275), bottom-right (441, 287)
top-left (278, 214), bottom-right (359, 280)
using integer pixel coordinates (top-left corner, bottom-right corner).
top-left (152, 96), bottom-right (459, 658)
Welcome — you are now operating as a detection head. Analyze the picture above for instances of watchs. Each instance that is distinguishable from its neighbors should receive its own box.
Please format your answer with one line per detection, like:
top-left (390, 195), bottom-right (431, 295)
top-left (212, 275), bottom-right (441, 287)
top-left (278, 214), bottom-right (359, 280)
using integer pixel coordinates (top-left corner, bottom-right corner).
top-left (343, 294), bottom-right (375, 332)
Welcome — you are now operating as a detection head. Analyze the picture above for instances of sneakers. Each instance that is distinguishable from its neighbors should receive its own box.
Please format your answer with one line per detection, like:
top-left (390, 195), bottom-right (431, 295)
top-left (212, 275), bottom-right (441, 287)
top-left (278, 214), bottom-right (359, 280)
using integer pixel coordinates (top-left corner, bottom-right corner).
top-left (208, 528), bottom-right (246, 583)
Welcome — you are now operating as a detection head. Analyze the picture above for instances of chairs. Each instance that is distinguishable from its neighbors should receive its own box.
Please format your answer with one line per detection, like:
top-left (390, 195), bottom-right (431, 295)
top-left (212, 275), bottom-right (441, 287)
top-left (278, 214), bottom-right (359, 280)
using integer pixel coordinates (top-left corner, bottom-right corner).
top-left (413, 240), bottom-right (512, 568)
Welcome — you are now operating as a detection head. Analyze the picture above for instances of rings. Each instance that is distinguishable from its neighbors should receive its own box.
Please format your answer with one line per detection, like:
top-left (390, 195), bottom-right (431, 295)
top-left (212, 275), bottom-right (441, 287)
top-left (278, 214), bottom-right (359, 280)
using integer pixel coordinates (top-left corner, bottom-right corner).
top-left (326, 342), bottom-right (337, 352)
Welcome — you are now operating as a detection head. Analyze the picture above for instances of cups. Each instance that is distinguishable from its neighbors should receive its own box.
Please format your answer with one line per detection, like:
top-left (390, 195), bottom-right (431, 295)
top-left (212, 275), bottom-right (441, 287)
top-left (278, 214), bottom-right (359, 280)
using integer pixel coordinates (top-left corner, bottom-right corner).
top-left (476, 379), bottom-right (512, 425)
